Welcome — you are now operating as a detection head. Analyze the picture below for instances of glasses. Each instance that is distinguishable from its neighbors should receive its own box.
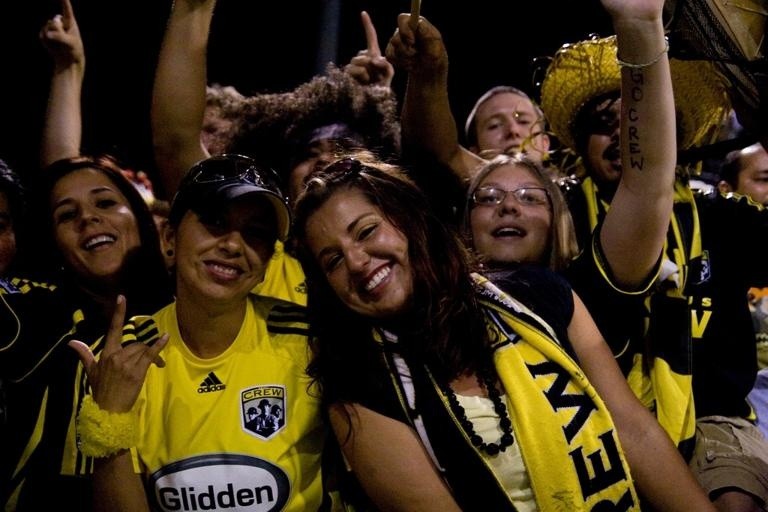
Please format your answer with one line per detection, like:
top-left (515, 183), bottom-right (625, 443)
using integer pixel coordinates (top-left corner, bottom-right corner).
top-left (471, 185), bottom-right (546, 208)
top-left (190, 155), bottom-right (286, 194)
top-left (323, 157), bottom-right (355, 180)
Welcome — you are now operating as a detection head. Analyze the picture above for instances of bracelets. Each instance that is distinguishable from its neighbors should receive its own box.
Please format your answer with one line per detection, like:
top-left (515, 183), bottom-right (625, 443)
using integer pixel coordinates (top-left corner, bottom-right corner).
top-left (616, 36), bottom-right (672, 68)
top-left (75, 394), bottom-right (140, 459)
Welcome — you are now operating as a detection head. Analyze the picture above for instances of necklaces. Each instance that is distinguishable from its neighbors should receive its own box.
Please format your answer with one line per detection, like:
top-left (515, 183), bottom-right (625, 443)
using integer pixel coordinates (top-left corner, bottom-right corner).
top-left (444, 376), bottom-right (513, 455)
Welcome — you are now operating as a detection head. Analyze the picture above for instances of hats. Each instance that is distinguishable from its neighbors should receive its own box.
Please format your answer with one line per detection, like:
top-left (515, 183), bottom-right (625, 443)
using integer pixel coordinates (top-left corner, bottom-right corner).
top-left (539, 34), bottom-right (733, 151)
top-left (216, 183), bottom-right (291, 241)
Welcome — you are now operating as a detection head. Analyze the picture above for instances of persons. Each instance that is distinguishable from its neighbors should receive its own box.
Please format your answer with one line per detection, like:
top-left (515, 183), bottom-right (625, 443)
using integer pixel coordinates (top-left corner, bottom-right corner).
top-left (718, 142), bottom-right (768, 435)
top-left (37, 0), bottom-right (248, 232)
top-left (463, 0), bottom-right (678, 382)
top-left (0, 159), bottom-right (26, 278)
top-left (0, 160), bottom-right (174, 512)
top-left (383, 13), bottom-right (768, 512)
top-left (66, 153), bottom-right (374, 512)
top-left (149, 0), bottom-right (359, 253)
top-left (341, 10), bottom-right (551, 172)
top-left (294, 157), bottom-right (719, 512)
